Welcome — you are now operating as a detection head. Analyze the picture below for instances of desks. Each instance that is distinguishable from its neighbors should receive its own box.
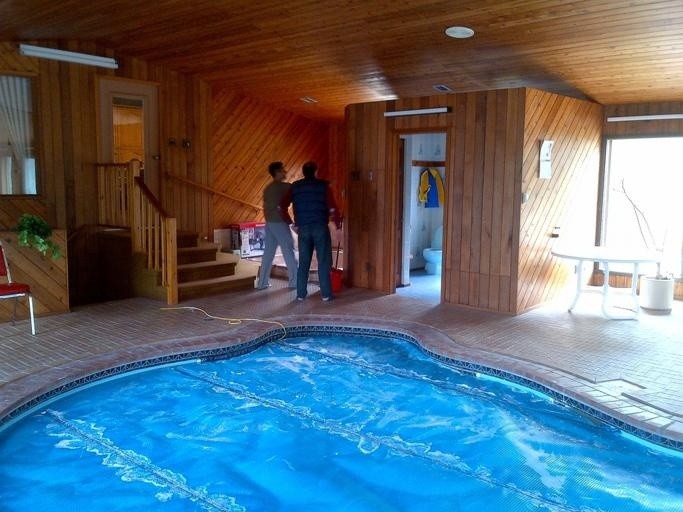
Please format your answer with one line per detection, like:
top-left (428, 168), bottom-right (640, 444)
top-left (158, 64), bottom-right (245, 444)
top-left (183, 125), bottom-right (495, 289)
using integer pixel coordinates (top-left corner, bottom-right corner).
top-left (552, 246), bottom-right (656, 314)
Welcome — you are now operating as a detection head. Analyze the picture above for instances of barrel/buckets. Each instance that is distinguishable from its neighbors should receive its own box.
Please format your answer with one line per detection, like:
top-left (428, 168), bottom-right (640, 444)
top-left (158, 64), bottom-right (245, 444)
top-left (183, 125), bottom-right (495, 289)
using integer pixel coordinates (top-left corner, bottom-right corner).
top-left (329, 270), bottom-right (344, 294)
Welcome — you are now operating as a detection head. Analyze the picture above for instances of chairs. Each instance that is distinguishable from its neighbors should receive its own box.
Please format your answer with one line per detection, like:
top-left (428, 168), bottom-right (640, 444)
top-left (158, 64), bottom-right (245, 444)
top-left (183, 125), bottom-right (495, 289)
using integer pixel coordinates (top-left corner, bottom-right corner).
top-left (0, 243), bottom-right (37, 335)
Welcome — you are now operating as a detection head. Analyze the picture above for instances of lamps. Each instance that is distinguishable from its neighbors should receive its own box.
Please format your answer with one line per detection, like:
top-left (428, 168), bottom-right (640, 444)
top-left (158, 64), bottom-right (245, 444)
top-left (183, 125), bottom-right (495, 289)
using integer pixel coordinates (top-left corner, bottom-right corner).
top-left (17, 43), bottom-right (120, 71)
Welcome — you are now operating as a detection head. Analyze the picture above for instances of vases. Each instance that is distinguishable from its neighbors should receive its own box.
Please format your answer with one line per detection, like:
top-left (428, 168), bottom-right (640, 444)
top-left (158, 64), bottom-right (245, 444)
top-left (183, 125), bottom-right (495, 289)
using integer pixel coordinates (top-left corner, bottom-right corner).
top-left (639, 276), bottom-right (674, 312)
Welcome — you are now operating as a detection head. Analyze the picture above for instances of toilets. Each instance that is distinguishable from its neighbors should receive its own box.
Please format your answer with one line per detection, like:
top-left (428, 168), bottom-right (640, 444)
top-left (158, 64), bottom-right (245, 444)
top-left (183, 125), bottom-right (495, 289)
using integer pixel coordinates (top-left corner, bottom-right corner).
top-left (423, 224), bottom-right (443, 274)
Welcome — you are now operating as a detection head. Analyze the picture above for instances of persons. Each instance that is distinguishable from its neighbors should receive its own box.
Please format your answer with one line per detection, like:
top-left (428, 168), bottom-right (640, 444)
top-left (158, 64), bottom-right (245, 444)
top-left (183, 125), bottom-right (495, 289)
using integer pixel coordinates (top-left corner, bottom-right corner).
top-left (277, 161), bottom-right (340, 302)
top-left (255, 161), bottom-right (308, 290)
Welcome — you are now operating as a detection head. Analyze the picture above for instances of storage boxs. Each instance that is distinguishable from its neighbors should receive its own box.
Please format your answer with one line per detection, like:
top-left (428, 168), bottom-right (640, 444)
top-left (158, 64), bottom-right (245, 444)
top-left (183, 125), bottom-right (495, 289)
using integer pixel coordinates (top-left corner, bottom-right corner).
top-left (230, 223), bottom-right (266, 259)
